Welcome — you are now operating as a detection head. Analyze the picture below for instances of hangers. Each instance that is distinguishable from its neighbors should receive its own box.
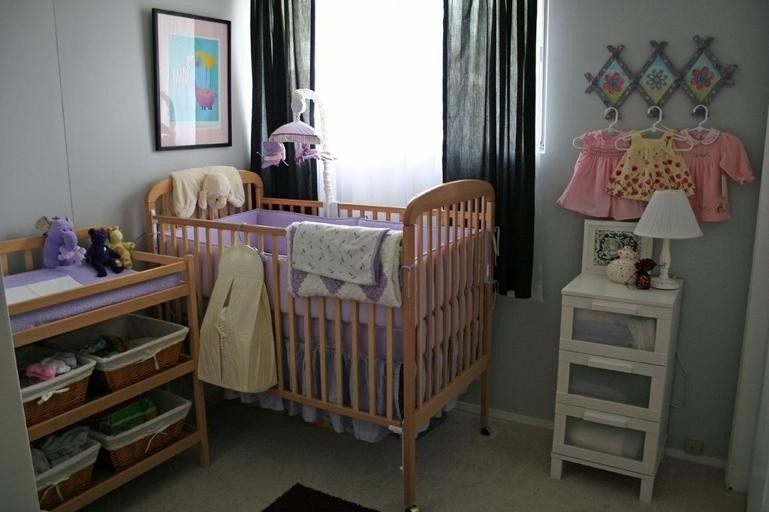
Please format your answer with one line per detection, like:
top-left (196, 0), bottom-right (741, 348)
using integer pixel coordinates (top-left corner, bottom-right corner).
top-left (687, 104), bottom-right (710, 134)
top-left (615, 106), bottom-right (694, 151)
top-left (572, 106), bottom-right (647, 152)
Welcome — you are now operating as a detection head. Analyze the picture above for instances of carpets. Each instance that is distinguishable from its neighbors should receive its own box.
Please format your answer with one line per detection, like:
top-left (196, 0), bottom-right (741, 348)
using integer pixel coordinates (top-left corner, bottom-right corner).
top-left (261, 483), bottom-right (384, 512)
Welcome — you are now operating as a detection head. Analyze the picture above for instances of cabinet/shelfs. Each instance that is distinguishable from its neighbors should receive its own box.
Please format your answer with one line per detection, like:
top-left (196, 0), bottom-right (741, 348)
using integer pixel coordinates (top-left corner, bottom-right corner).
top-left (0, 225), bottom-right (211, 512)
top-left (550, 271), bottom-right (685, 503)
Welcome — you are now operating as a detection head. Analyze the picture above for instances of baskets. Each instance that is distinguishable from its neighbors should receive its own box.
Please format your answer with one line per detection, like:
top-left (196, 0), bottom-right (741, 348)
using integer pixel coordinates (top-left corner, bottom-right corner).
top-left (42, 315), bottom-right (190, 395)
top-left (87, 390), bottom-right (193, 472)
top-left (15, 346), bottom-right (96, 428)
top-left (28, 436), bottom-right (103, 512)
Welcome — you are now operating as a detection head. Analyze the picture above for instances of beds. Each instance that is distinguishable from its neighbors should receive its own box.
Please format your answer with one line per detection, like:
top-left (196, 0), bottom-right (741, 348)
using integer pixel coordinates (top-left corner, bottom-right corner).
top-left (143, 169), bottom-right (496, 511)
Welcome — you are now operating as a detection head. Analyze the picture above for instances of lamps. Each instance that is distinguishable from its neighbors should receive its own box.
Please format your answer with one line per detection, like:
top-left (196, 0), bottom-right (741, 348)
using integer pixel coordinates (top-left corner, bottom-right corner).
top-left (269, 88), bottom-right (335, 218)
top-left (633, 188), bottom-right (704, 290)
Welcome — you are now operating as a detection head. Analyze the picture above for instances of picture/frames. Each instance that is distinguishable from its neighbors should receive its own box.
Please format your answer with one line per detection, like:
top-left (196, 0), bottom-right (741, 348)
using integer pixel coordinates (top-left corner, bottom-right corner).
top-left (680, 35), bottom-right (738, 117)
top-left (150, 7), bottom-right (233, 152)
top-left (585, 44), bottom-right (637, 121)
top-left (637, 41), bottom-right (680, 119)
top-left (581, 218), bottom-right (653, 275)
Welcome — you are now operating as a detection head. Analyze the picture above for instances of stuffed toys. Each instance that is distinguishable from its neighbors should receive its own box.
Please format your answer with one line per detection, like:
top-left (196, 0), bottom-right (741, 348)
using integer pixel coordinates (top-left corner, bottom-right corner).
top-left (107, 225), bottom-right (135, 269)
top-left (33, 215), bottom-right (87, 269)
top-left (198, 172), bottom-right (234, 211)
top-left (85, 227), bottom-right (124, 278)
